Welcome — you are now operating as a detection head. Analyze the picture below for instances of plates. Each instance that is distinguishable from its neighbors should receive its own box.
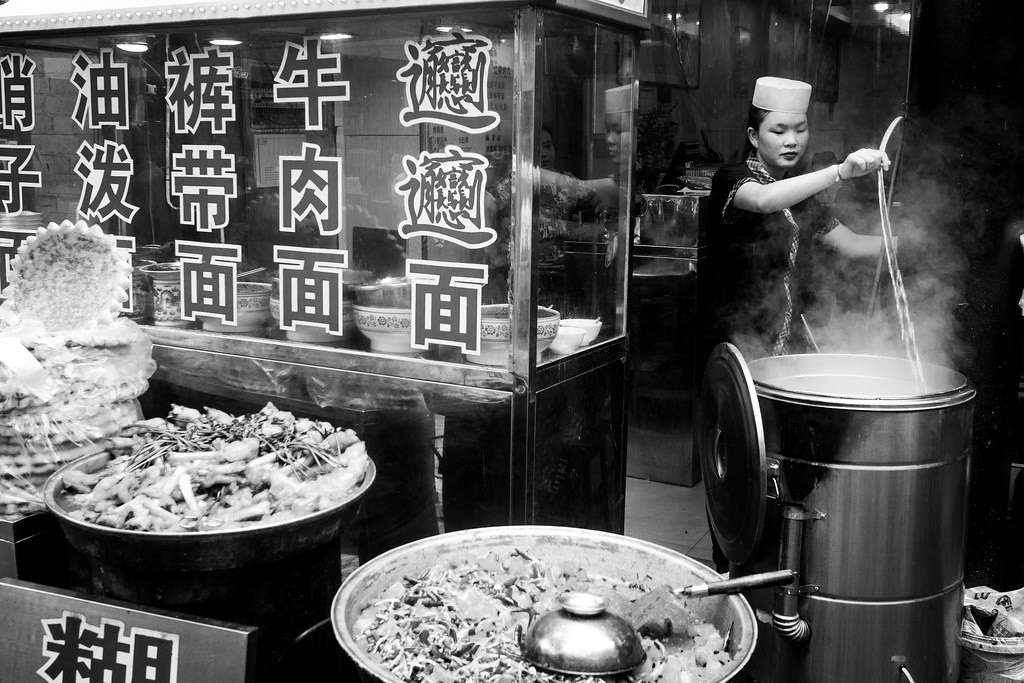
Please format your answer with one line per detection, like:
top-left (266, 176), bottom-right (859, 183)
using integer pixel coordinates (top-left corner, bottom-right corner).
top-left (132, 259), bottom-right (183, 280)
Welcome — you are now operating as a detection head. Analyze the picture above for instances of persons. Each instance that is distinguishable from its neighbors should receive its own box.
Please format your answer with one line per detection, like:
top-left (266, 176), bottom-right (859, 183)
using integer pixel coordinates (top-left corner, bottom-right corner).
top-left (477, 123), bottom-right (581, 319)
top-left (532, 80), bottom-right (640, 337)
top-left (708, 76), bottom-right (897, 580)
top-left (131, 138), bottom-right (174, 246)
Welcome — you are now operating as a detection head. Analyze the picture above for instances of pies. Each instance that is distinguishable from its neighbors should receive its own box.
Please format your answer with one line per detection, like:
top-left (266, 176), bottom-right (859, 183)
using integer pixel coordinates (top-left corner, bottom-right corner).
top-left (0, 219), bottom-right (149, 514)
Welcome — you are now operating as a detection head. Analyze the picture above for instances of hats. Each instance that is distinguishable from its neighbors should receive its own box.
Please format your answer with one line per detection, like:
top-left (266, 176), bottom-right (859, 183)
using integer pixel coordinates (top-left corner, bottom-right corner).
top-left (605, 85), bottom-right (632, 114)
top-left (752, 77), bottom-right (812, 114)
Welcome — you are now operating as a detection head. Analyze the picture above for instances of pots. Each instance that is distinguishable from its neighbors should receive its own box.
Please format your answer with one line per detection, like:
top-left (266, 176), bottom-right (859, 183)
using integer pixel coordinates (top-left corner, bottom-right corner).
top-left (40, 441), bottom-right (377, 603)
top-left (330, 523), bottom-right (760, 683)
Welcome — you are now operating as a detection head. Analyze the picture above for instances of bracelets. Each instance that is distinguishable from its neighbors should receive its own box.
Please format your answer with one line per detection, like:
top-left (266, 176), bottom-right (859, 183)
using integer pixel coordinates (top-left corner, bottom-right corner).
top-left (836, 164), bottom-right (844, 183)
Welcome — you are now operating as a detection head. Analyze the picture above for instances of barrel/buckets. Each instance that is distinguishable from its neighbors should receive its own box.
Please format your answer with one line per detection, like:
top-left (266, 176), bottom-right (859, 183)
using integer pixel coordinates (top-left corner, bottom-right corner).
top-left (717, 351), bottom-right (979, 682)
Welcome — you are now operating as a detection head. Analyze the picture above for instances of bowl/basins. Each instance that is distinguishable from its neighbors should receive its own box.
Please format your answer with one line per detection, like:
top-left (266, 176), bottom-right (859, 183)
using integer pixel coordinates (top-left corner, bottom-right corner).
top-left (460, 303), bottom-right (560, 366)
top-left (352, 303), bottom-right (436, 354)
top-left (546, 327), bottom-right (585, 354)
top-left (269, 296), bottom-right (355, 343)
top-left (521, 591), bottom-right (648, 678)
top-left (196, 281), bottom-right (272, 332)
top-left (562, 319), bottom-right (602, 346)
